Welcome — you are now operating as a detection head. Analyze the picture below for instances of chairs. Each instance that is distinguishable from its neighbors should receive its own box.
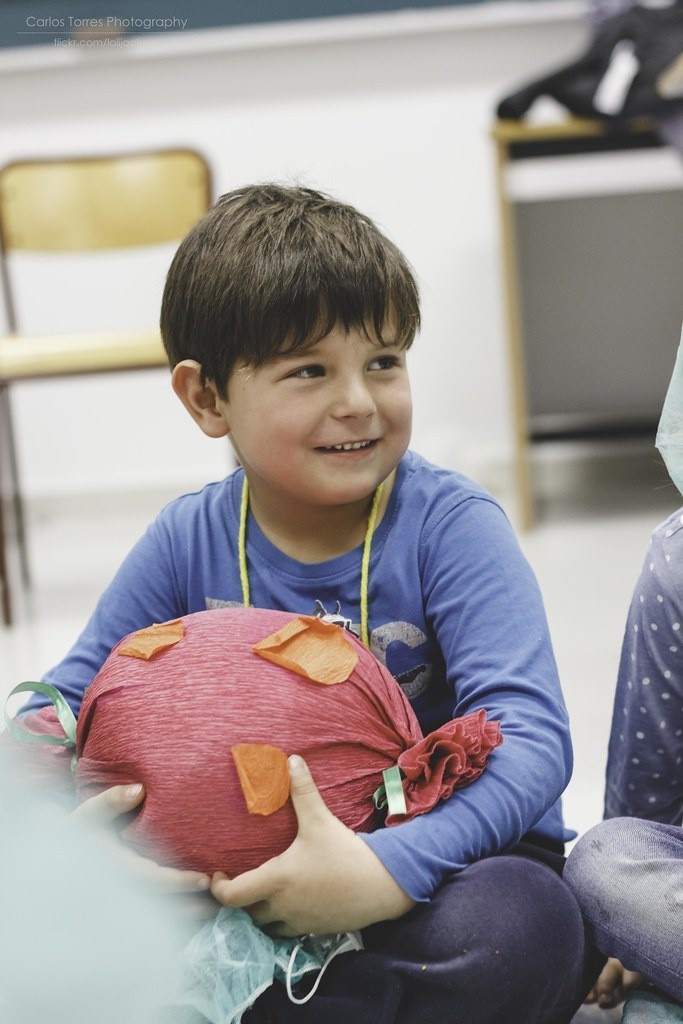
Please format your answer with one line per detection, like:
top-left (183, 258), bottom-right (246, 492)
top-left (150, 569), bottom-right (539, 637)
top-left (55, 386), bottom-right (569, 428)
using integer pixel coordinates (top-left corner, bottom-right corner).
top-left (0, 147), bottom-right (239, 627)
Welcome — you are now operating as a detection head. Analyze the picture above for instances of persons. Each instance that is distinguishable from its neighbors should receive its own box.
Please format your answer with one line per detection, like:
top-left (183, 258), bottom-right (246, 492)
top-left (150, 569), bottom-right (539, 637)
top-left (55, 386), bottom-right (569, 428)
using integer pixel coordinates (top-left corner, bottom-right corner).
top-left (563, 331), bottom-right (683, 1008)
top-left (6, 184), bottom-right (586, 1024)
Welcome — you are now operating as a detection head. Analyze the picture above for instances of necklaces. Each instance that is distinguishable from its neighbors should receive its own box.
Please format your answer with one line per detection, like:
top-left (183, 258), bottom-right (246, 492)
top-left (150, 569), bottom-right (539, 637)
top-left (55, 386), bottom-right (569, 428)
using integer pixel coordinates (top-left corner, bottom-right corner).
top-left (238, 474), bottom-right (384, 650)
